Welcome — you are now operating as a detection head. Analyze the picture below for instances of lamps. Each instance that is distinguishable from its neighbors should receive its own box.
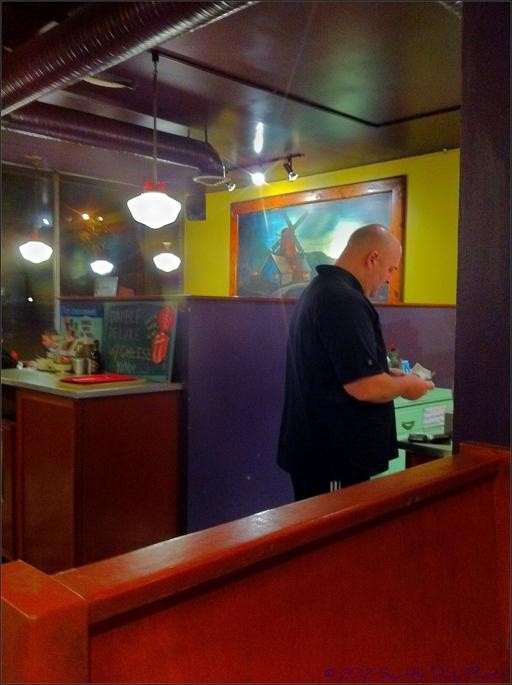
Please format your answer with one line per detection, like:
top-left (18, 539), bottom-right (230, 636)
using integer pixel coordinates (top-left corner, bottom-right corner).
top-left (89, 259), bottom-right (114, 275)
top-left (80, 212), bottom-right (90, 220)
top-left (18, 240), bottom-right (54, 263)
top-left (126, 54), bottom-right (182, 230)
top-left (98, 215), bottom-right (105, 222)
top-left (153, 240), bottom-right (182, 273)
top-left (225, 182), bottom-right (236, 192)
top-left (283, 162), bottom-right (299, 183)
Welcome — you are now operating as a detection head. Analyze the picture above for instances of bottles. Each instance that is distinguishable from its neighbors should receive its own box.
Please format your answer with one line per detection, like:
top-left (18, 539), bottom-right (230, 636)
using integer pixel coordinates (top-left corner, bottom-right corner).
top-left (91, 340), bottom-right (101, 374)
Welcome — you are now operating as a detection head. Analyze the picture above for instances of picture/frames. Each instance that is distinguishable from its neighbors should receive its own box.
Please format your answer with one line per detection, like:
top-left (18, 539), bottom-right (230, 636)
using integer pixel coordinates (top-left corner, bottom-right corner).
top-left (229, 174), bottom-right (407, 304)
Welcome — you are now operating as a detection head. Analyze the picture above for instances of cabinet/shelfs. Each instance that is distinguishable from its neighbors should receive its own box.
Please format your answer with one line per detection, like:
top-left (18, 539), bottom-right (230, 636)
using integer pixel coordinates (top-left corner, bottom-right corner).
top-left (0, 366), bottom-right (184, 576)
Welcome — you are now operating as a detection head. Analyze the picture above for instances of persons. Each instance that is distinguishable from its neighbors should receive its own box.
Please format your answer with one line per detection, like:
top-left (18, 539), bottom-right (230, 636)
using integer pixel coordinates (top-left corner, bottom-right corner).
top-left (23, 329), bottom-right (57, 367)
top-left (276, 224), bottom-right (435, 502)
